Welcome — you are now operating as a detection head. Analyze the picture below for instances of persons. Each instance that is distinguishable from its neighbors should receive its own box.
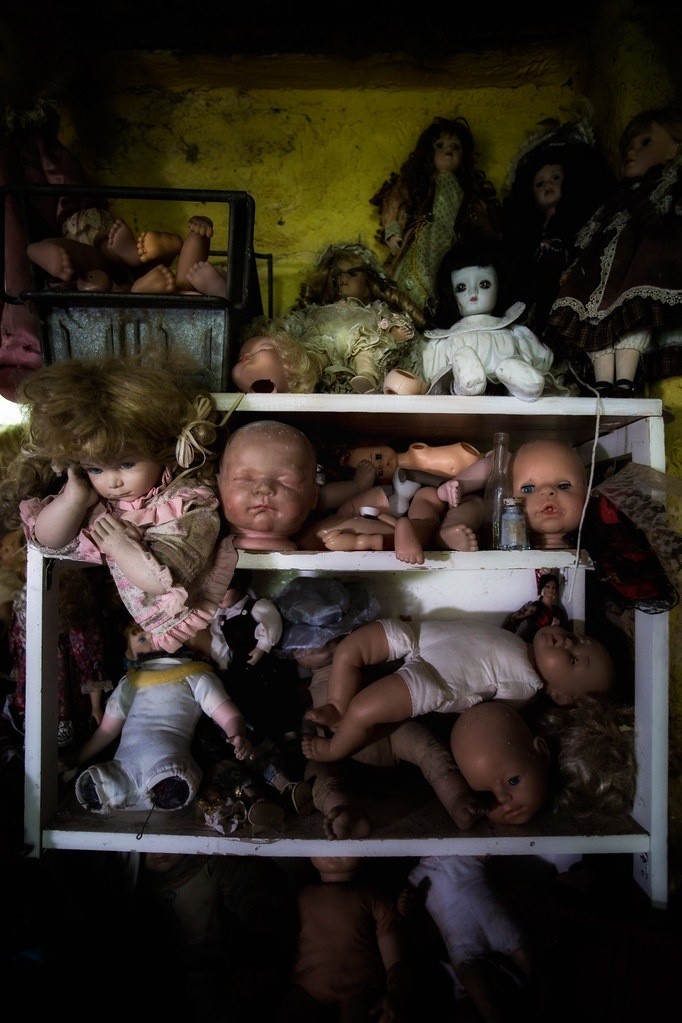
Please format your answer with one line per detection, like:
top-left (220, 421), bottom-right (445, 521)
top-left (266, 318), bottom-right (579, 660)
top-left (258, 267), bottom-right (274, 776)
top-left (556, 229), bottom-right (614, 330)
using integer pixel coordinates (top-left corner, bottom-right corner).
top-left (2, 83), bottom-right (682, 1021)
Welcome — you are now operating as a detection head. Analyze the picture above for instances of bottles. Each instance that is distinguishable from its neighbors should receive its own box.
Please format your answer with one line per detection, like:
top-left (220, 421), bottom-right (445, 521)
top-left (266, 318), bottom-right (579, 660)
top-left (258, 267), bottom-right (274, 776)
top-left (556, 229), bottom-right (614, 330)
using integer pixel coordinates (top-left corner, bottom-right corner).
top-left (483, 432), bottom-right (512, 549)
top-left (499, 497), bottom-right (532, 550)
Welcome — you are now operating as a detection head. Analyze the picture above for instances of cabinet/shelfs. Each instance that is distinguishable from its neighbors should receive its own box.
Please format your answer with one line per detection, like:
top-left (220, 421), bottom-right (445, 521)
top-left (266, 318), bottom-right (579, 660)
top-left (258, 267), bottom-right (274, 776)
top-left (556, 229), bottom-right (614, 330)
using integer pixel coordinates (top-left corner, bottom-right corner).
top-left (21, 389), bottom-right (671, 917)
top-left (15, 179), bottom-right (257, 390)
